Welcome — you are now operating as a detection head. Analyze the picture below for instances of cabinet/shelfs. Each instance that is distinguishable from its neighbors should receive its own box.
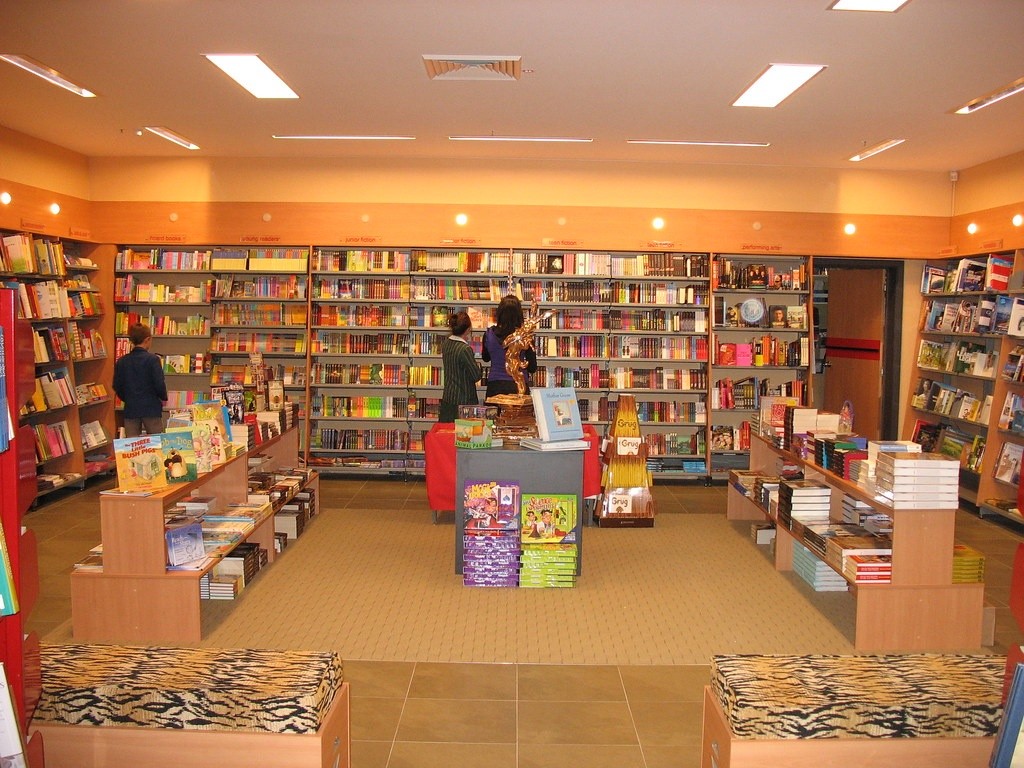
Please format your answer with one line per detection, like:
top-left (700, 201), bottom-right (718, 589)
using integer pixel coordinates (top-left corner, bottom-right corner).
top-left (69, 425), bottom-right (320, 645)
top-left (0, 226), bottom-right (117, 512)
top-left (813, 274), bottom-right (828, 374)
top-left (726, 431), bottom-right (995, 653)
top-left (592, 393), bottom-right (655, 528)
top-left (455, 444), bottom-right (585, 576)
top-left (0, 287), bottom-right (47, 768)
top-left (115, 244), bottom-right (813, 487)
top-left (1002, 450), bottom-right (1024, 708)
top-left (901, 248), bottom-right (1024, 525)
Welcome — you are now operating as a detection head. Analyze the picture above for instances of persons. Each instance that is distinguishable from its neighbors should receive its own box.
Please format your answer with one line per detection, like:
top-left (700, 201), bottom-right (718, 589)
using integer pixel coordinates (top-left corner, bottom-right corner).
top-left (774, 275), bottom-right (782, 290)
top-left (112, 323), bottom-right (168, 438)
top-left (726, 307), bottom-right (736, 319)
top-left (772, 306), bottom-right (784, 322)
top-left (715, 433), bottom-right (727, 451)
top-left (482, 295), bottom-right (538, 400)
top-left (438, 312), bottom-right (483, 422)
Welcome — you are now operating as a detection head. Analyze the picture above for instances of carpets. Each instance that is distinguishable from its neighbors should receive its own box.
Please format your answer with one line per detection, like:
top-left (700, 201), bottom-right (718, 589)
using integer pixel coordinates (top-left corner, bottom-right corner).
top-left (205, 508), bottom-right (855, 666)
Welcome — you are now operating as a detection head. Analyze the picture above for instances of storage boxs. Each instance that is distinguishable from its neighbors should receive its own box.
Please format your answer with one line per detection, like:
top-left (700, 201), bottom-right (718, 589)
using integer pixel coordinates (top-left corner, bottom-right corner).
top-left (455, 418), bottom-right (493, 449)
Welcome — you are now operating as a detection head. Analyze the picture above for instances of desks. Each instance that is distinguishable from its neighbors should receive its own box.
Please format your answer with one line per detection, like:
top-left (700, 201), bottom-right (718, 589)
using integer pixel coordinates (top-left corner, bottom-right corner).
top-left (425, 423), bottom-right (602, 528)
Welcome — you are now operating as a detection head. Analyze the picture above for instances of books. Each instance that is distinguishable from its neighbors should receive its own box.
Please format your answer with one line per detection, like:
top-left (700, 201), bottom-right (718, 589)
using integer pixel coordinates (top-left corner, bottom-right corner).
top-left (728, 396), bottom-right (984, 591)
top-left (911, 254), bottom-right (1024, 517)
top-left (113, 247), bottom-right (809, 473)
top-left (0, 326), bottom-right (30, 768)
top-left (461, 480), bottom-right (577, 588)
top-left (0, 231), bottom-right (111, 492)
top-left (74, 400), bottom-right (315, 601)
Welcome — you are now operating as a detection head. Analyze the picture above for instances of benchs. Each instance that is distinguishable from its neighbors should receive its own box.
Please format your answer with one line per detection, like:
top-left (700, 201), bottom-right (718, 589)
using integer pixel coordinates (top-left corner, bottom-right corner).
top-left (28, 641), bottom-right (351, 768)
top-left (701, 653), bottom-right (1007, 768)
